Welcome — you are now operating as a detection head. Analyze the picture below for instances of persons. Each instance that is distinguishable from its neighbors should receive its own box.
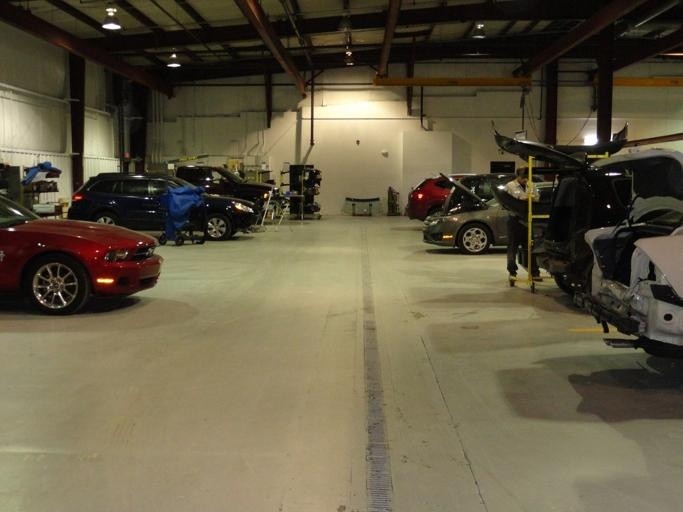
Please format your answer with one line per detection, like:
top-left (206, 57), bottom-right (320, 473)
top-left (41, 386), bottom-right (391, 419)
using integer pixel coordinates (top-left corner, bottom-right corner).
top-left (503, 168), bottom-right (542, 282)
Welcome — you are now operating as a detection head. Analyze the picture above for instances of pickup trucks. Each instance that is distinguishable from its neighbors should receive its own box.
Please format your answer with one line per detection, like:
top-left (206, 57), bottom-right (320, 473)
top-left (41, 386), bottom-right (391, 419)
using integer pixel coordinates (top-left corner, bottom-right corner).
top-left (174, 165), bottom-right (279, 226)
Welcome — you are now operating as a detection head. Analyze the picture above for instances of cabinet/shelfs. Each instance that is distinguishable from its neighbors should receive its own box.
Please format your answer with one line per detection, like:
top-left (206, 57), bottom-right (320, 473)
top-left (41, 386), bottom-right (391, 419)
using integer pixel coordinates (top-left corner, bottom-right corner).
top-left (288, 163), bottom-right (320, 221)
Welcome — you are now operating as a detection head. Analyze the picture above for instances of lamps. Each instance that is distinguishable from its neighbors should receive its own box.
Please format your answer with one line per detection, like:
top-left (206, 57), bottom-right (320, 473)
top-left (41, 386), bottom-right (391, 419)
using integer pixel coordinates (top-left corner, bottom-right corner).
top-left (472, 26), bottom-right (486, 39)
top-left (343, 48), bottom-right (354, 68)
top-left (162, 51), bottom-right (181, 69)
top-left (100, 1), bottom-right (124, 34)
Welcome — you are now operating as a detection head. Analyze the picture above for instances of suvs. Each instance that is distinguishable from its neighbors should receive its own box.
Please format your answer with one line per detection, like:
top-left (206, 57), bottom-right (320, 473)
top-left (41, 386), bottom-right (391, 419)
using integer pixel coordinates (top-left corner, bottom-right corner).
top-left (66, 172), bottom-right (256, 244)
top-left (443, 174), bottom-right (544, 215)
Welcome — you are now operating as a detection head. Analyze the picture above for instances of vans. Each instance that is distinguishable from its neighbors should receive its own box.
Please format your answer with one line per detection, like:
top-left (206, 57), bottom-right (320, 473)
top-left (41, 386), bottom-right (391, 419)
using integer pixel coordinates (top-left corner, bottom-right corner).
top-left (493, 128), bottom-right (683, 295)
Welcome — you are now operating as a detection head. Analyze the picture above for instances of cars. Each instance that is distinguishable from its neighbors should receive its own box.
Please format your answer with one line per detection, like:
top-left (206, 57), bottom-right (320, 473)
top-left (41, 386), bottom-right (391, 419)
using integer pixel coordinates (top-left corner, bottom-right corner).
top-left (406, 171), bottom-right (479, 223)
top-left (582, 213), bottom-right (683, 357)
top-left (0, 194), bottom-right (163, 314)
top-left (421, 173), bottom-right (557, 255)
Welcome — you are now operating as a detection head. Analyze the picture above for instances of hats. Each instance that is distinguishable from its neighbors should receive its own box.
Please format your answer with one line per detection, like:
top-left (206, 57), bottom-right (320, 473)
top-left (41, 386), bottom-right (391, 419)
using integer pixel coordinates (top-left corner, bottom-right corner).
top-left (517, 164), bottom-right (529, 178)
